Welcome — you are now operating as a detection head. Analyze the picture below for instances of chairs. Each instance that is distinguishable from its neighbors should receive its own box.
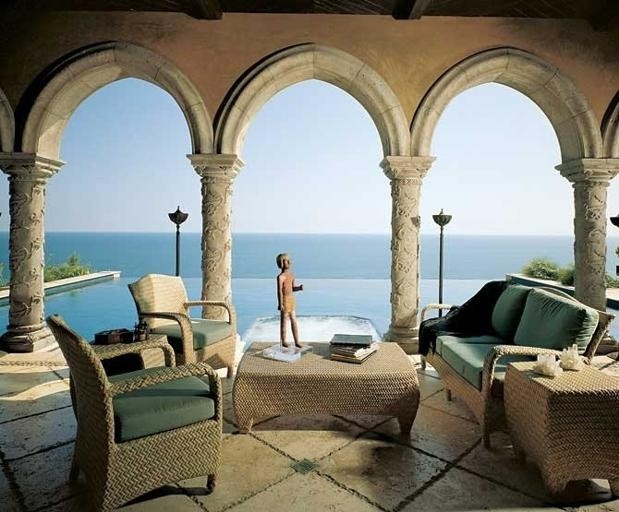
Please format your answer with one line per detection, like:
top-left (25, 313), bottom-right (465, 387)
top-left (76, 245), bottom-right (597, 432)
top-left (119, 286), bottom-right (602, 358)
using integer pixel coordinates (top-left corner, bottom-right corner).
top-left (46, 314), bottom-right (223, 511)
top-left (129, 273), bottom-right (236, 381)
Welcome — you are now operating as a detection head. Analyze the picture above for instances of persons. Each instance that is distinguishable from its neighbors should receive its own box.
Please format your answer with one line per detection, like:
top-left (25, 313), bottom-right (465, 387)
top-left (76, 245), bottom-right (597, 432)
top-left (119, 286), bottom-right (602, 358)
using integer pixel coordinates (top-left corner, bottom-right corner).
top-left (276, 252), bottom-right (303, 349)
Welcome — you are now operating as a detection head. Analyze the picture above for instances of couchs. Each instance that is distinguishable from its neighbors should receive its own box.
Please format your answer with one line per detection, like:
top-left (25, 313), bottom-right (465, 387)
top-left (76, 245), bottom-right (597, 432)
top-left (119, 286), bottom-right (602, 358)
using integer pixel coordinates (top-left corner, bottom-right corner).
top-left (418, 280), bottom-right (615, 450)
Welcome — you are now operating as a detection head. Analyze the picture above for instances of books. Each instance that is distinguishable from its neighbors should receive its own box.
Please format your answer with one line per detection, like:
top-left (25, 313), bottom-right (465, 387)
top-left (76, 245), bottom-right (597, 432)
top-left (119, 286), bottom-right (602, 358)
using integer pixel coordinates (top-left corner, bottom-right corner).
top-left (328, 333), bottom-right (377, 364)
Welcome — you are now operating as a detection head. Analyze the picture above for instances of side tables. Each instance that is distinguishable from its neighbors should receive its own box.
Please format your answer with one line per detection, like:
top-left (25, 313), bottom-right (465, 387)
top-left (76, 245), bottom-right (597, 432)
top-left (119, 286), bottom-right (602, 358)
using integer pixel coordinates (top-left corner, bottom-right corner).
top-left (504, 358), bottom-right (619, 501)
top-left (68, 332), bottom-right (176, 424)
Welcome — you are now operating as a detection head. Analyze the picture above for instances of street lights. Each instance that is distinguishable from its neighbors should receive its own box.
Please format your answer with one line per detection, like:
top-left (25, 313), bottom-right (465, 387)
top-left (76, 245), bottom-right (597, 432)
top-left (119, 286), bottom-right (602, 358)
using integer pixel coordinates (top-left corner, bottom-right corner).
top-left (168, 205), bottom-right (189, 276)
top-left (432, 208), bottom-right (452, 317)
